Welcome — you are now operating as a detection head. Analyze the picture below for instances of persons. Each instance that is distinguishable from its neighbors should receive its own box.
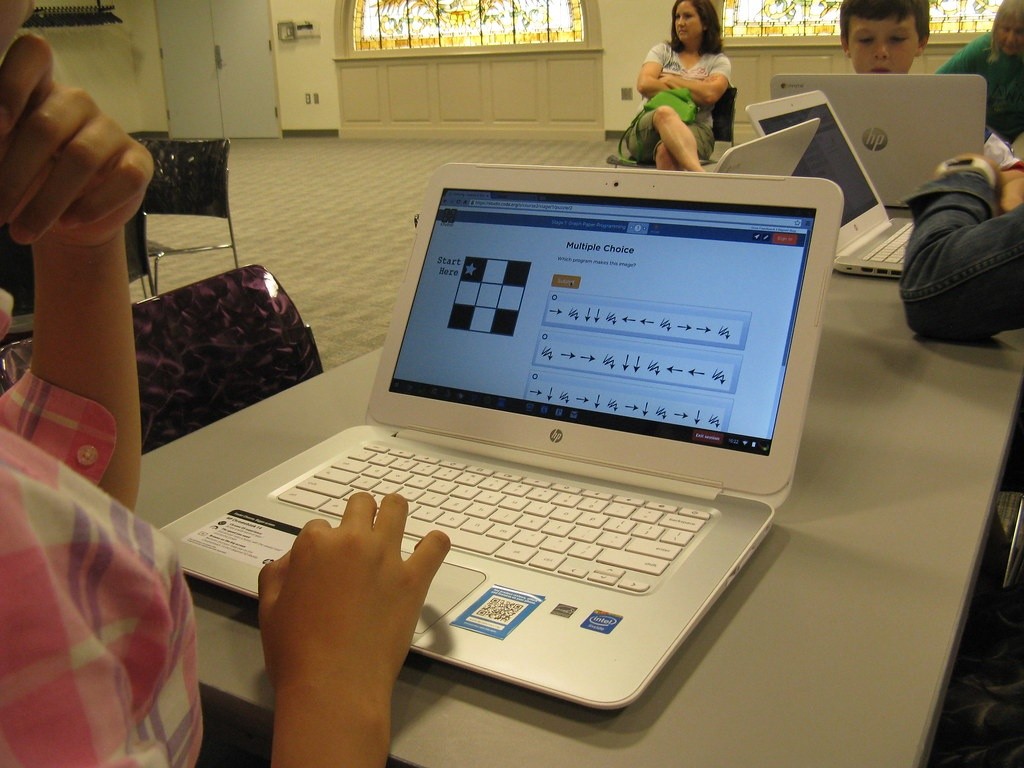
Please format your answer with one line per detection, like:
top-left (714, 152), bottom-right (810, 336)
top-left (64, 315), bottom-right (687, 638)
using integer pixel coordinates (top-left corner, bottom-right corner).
top-left (932, 0), bottom-right (1024, 144)
top-left (899, 153), bottom-right (1024, 342)
top-left (626, 0), bottom-right (731, 172)
top-left (0, 0), bottom-right (450, 768)
top-left (839, 0), bottom-right (1024, 213)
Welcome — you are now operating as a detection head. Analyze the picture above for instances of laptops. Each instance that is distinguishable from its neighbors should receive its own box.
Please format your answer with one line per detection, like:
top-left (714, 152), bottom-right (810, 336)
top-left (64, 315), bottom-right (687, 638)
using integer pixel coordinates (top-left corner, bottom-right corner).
top-left (150, 74), bottom-right (988, 710)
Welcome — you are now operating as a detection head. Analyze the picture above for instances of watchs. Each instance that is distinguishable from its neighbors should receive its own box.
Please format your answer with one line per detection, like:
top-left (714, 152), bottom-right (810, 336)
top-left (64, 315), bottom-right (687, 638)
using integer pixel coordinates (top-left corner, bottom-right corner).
top-left (937, 156), bottom-right (996, 186)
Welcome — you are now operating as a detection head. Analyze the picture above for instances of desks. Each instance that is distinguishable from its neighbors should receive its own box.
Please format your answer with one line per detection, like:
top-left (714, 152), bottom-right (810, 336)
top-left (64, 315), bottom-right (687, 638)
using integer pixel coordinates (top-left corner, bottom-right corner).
top-left (136, 204), bottom-right (1024, 768)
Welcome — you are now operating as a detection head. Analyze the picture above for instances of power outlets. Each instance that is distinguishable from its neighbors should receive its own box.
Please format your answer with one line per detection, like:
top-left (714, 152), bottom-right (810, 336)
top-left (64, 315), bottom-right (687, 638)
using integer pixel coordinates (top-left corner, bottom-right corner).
top-left (306, 93), bottom-right (311, 104)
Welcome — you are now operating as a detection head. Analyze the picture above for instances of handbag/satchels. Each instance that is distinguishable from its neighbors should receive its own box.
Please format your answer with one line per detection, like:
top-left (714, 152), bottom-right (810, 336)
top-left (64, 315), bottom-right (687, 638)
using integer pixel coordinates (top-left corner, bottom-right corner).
top-left (618, 87), bottom-right (700, 165)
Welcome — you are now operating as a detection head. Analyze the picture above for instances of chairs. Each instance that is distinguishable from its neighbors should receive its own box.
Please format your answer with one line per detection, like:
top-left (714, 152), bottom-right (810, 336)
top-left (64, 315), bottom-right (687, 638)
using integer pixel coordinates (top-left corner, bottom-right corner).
top-left (628, 86), bottom-right (737, 170)
top-left (0, 210), bottom-right (156, 316)
top-left (0, 264), bottom-right (323, 454)
top-left (136, 134), bottom-right (242, 293)
